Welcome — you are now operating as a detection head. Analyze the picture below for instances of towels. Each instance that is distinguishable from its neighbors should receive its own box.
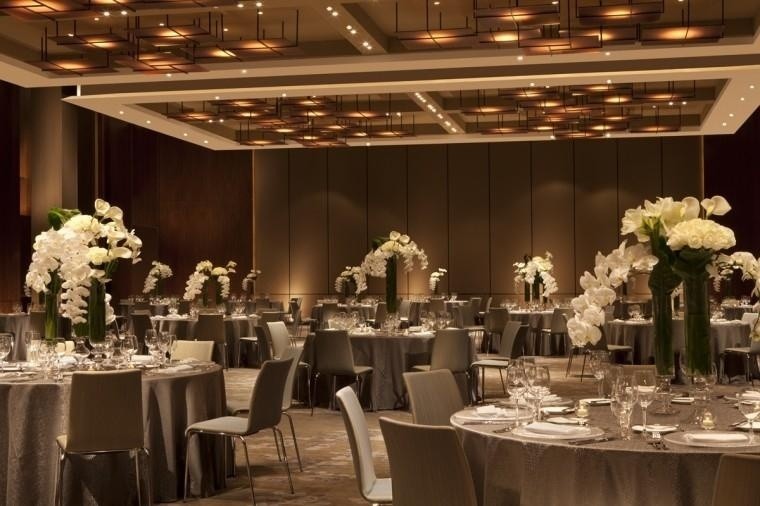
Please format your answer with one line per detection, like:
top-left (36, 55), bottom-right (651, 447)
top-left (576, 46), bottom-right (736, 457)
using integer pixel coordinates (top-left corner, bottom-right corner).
top-left (183, 357), bottom-right (199, 364)
top-left (477, 405), bottom-right (505, 417)
top-left (167, 365), bottom-right (192, 372)
top-left (685, 433), bottom-right (747, 442)
top-left (525, 422), bottom-right (589, 435)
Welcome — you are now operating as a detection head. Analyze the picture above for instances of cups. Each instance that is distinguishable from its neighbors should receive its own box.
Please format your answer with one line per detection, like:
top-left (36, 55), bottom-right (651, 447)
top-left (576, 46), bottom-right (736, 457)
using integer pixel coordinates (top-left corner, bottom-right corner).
top-left (428, 312), bottom-right (435, 329)
top-left (145, 329), bottom-right (157, 354)
top-left (28, 333), bottom-right (41, 365)
top-left (615, 375), bottom-right (638, 416)
top-left (105, 331), bottom-right (114, 367)
top-left (589, 351), bottom-right (608, 398)
top-left (120, 339), bottom-right (134, 369)
top-left (610, 384), bottom-right (632, 441)
top-left (521, 358), bottom-right (536, 392)
top-left (421, 311), bottom-right (427, 325)
top-left (506, 359), bottom-right (525, 428)
top-left (633, 370), bottom-right (656, 442)
top-left (0, 336), bottom-right (11, 374)
top-left (738, 398), bottom-right (760, 445)
top-left (527, 366), bottom-right (550, 423)
top-left (167, 335), bottom-right (177, 368)
top-left (38, 341), bottom-right (54, 382)
top-left (52, 338), bottom-right (66, 382)
top-left (125, 335), bottom-right (138, 365)
top-left (158, 332), bottom-right (168, 362)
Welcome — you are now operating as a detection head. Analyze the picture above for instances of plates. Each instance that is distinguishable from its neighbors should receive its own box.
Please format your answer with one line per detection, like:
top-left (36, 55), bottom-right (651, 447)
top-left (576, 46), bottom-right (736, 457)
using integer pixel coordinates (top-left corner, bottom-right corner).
top-left (580, 398), bottom-right (615, 405)
top-left (689, 432), bottom-right (747, 442)
top-left (454, 408), bottom-right (534, 421)
top-left (631, 424), bottom-right (677, 434)
top-left (664, 431), bottom-right (760, 449)
top-left (510, 426), bottom-right (605, 441)
top-left (525, 422), bottom-right (577, 435)
top-left (733, 421), bottom-right (760, 431)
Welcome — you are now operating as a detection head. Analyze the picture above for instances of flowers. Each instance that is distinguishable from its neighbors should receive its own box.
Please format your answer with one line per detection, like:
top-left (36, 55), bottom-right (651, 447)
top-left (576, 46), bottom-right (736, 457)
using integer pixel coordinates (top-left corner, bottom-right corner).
top-left (514, 250), bottom-right (558, 305)
top-left (143, 260), bottom-right (172, 298)
top-left (568, 241), bottom-right (760, 374)
top-left (184, 271), bottom-right (209, 305)
top-left (335, 231), bottom-right (428, 312)
top-left (197, 260), bottom-right (237, 304)
top-left (24, 199), bottom-right (143, 346)
top-left (429, 268), bottom-right (447, 294)
top-left (707, 251), bottom-right (759, 318)
top-left (623, 193), bottom-right (736, 373)
top-left (243, 269), bottom-right (261, 300)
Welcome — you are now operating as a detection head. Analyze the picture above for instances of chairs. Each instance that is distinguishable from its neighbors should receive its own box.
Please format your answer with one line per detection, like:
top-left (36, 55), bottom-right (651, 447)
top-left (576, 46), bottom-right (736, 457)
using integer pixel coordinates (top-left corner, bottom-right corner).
top-left (315, 330), bottom-right (373, 411)
top-left (227, 347), bottom-right (304, 472)
top-left (429, 300), bottom-right (444, 316)
top-left (542, 307), bottom-right (573, 356)
top-left (405, 368), bottom-right (463, 425)
top-left (283, 310), bottom-right (302, 348)
top-left (464, 297), bottom-right (481, 325)
top-left (581, 324), bottom-right (632, 383)
top-left (183, 360), bottom-right (294, 504)
top-left (474, 320), bottom-right (521, 399)
top-left (54, 369), bottom-right (151, 506)
top-left (336, 385), bottom-right (393, 505)
top-left (489, 308), bottom-right (530, 355)
top-left (407, 329), bottom-right (469, 407)
top-left (178, 301), bottom-right (190, 314)
top-left (319, 302), bottom-right (338, 329)
top-left (400, 301), bottom-right (420, 328)
top-left (111, 316), bottom-right (139, 355)
top-left (237, 311), bottom-right (281, 367)
top-left (265, 322), bottom-right (291, 361)
top-left (366, 303), bottom-right (387, 328)
top-left (150, 305), bottom-right (166, 316)
top-left (171, 340), bottom-right (215, 362)
top-left (120, 301), bottom-right (149, 349)
top-left (255, 298), bottom-right (271, 311)
top-left (27, 310), bottom-right (58, 342)
top-left (380, 417), bottom-right (477, 506)
top-left (131, 313), bottom-right (158, 349)
top-left (468, 335), bottom-right (508, 403)
top-left (670, 318), bottom-right (686, 375)
top-left (447, 301), bottom-right (452, 314)
top-left (289, 301), bottom-right (317, 331)
top-left (272, 300), bottom-right (284, 311)
top-left (284, 298), bottom-right (303, 317)
top-left (448, 293), bottom-right (457, 301)
top-left (241, 301), bottom-right (255, 312)
top-left (724, 311), bottom-right (760, 384)
top-left (741, 312), bottom-right (758, 338)
top-left (254, 325), bottom-right (311, 409)
top-left (479, 296), bottom-right (492, 318)
top-left (197, 314), bottom-right (229, 372)
top-left (285, 298), bottom-right (298, 314)
top-left (621, 363), bottom-right (657, 387)
top-left (453, 306), bottom-right (488, 353)
